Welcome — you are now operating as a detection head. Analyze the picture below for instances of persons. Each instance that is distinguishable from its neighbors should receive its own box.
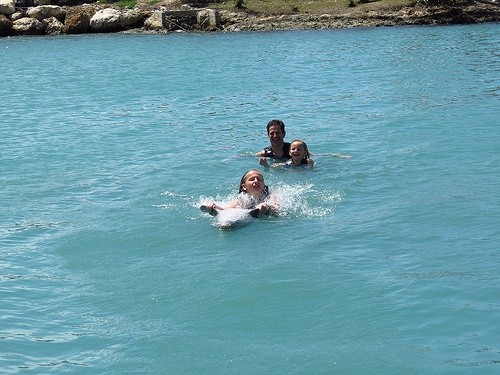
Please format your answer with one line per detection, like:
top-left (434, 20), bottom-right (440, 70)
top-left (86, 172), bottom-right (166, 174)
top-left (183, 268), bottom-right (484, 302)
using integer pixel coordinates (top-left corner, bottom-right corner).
top-left (200, 169), bottom-right (280, 219)
top-left (258, 139), bottom-right (314, 170)
top-left (255, 119), bottom-right (312, 158)
